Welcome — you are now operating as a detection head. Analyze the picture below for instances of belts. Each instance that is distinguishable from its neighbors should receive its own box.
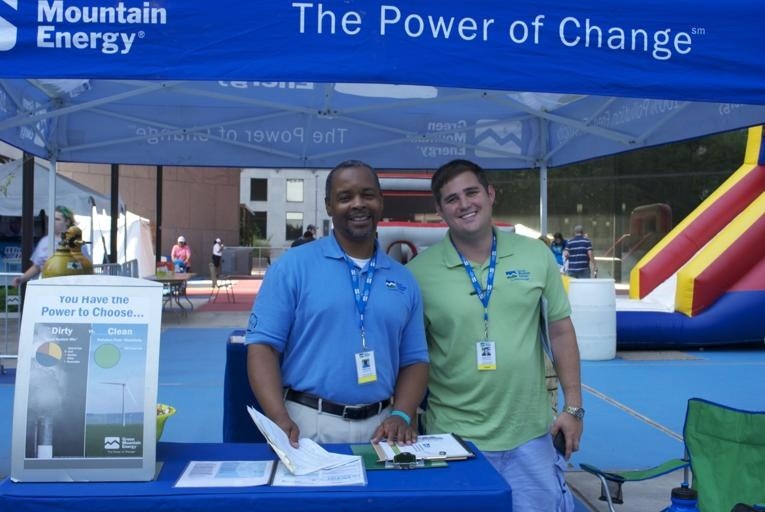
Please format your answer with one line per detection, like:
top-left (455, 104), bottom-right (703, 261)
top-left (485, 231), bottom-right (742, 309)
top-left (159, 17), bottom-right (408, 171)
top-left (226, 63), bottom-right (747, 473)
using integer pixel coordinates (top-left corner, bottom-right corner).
top-left (284, 390), bottom-right (390, 421)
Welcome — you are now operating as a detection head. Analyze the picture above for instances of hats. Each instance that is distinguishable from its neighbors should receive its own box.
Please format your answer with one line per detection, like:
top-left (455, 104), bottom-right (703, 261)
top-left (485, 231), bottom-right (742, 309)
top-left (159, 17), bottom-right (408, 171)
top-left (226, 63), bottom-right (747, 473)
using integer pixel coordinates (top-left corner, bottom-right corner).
top-left (178, 235), bottom-right (186, 243)
top-left (216, 237), bottom-right (222, 243)
top-left (307, 223), bottom-right (319, 230)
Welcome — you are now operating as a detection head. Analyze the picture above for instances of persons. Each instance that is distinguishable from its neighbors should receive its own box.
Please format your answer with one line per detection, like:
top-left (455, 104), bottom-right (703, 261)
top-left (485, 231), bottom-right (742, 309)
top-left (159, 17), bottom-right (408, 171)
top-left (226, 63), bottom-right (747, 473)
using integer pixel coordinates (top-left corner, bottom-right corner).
top-left (11, 205), bottom-right (93, 288)
top-left (211, 236), bottom-right (225, 289)
top-left (550, 231), bottom-right (568, 272)
top-left (405, 159), bottom-right (585, 512)
top-left (562, 224), bottom-right (596, 279)
top-left (243, 159), bottom-right (432, 450)
top-left (290, 230), bottom-right (317, 248)
top-left (170, 236), bottom-right (193, 298)
top-left (298, 224), bottom-right (319, 241)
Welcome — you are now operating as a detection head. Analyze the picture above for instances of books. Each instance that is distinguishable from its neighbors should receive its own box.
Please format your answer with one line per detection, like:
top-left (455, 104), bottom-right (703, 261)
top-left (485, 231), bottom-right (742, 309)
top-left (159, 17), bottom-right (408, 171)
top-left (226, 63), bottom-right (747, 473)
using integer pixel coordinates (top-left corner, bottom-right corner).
top-left (172, 460), bottom-right (367, 489)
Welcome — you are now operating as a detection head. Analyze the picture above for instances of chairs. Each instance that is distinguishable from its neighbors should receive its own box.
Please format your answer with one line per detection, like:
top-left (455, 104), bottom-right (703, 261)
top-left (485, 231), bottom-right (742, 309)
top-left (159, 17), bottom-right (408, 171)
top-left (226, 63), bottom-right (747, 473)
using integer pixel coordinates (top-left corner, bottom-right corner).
top-left (208, 262), bottom-right (235, 304)
top-left (579, 398), bottom-right (765, 512)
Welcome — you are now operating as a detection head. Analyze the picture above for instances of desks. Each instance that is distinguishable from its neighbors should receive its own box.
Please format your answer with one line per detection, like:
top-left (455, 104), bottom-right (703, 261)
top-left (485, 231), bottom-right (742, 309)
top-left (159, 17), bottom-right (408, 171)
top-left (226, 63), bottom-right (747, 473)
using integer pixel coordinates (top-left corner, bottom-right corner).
top-left (222, 331), bottom-right (270, 443)
top-left (0, 440), bottom-right (513, 512)
top-left (144, 272), bottom-right (198, 313)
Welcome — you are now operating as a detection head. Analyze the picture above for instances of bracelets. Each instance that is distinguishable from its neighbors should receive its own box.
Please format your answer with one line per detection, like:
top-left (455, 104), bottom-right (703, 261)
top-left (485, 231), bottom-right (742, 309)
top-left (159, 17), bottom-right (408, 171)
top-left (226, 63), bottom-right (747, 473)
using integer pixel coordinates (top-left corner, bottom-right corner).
top-left (390, 410), bottom-right (411, 426)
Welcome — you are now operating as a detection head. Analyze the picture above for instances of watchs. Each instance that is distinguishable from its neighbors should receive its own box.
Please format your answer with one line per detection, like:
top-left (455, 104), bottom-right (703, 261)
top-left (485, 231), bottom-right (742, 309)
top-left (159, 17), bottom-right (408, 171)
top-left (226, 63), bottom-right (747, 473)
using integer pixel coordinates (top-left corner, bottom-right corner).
top-left (561, 403), bottom-right (585, 421)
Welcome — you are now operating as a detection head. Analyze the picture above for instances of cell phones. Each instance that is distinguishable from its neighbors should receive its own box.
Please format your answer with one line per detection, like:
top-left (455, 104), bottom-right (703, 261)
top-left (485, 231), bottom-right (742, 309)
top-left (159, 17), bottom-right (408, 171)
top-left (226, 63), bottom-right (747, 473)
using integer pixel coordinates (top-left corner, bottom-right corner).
top-left (553, 429), bottom-right (566, 457)
